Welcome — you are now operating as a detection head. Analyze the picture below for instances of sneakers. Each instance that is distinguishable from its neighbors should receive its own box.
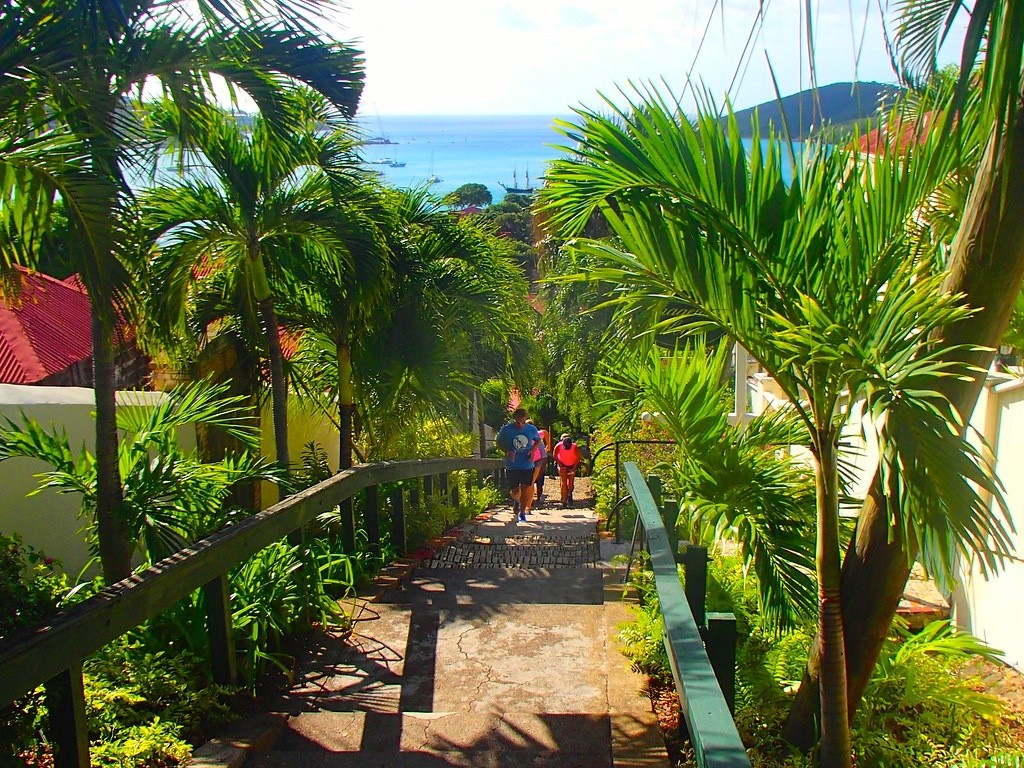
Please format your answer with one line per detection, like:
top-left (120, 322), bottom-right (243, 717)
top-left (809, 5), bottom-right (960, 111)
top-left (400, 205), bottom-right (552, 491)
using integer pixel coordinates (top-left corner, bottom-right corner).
top-left (517, 512), bottom-right (527, 523)
top-left (513, 500), bottom-right (520, 516)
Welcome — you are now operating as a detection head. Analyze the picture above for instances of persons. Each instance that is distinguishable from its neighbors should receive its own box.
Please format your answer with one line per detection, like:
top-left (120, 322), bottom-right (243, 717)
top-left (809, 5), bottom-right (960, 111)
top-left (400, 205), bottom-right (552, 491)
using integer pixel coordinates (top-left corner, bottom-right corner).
top-left (553, 437), bottom-right (581, 507)
top-left (497, 409), bottom-right (550, 521)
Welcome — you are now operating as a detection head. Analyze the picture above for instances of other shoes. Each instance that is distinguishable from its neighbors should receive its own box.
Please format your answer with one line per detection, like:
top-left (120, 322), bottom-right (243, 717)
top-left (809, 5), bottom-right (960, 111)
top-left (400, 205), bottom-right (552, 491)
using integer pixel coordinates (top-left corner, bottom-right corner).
top-left (567, 497), bottom-right (574, 508)
top-left (536, 501), bottom-right (543, 510)
top-left (561, 504), bottom-right (567, 509)
top-left (530, 504), bottom-right (534, 510)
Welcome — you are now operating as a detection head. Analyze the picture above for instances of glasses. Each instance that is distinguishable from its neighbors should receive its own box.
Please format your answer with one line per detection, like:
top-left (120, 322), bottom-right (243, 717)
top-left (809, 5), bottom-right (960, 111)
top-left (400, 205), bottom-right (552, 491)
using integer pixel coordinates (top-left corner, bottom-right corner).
top-left (515, 420), bottom-right (525, 423)
